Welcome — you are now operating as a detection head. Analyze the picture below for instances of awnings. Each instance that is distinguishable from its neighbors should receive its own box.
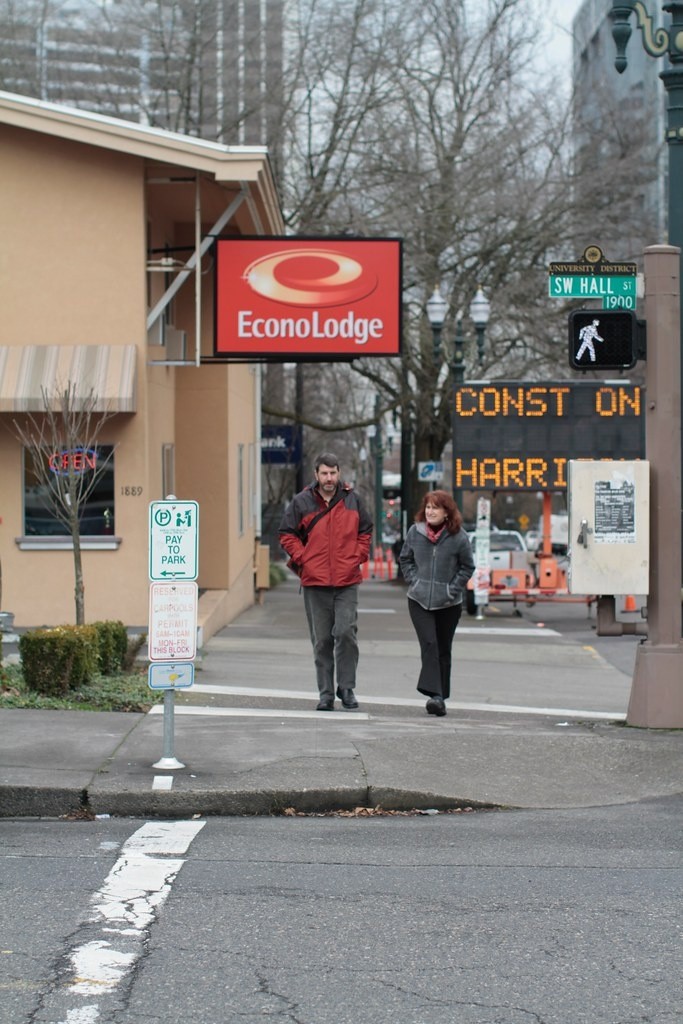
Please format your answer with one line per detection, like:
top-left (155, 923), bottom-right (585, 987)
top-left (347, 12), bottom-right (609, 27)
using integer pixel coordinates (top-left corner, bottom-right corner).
top-left (0, 345), bottom-right (137, 414)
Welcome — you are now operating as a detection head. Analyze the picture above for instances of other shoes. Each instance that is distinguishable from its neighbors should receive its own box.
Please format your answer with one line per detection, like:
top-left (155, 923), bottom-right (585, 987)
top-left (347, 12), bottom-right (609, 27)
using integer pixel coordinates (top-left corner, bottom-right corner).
top-left (426, 697), bottom-right (447, 715)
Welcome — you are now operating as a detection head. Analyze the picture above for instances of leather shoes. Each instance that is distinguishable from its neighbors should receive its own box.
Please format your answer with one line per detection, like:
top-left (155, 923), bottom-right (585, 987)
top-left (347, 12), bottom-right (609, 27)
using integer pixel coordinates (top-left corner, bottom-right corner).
top-left (336, 686), bottom-right (358, 708)
top-left (316, 700), bottom-right (334, 710)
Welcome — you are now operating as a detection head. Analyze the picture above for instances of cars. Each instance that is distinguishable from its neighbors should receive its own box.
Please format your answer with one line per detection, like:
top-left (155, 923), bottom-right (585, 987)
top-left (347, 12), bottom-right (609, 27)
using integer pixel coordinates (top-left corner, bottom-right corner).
top-left (463, 531), bottom-right (529, 615)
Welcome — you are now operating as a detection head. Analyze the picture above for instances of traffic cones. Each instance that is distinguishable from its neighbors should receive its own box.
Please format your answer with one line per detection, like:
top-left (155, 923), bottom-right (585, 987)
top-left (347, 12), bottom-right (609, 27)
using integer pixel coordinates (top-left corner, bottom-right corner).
top-left (620, 594), bottom-right (641, 613)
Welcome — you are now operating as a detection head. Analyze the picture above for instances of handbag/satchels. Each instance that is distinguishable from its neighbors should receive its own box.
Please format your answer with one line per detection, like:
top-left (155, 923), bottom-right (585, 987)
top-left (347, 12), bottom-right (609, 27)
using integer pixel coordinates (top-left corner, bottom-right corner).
top-left (287, 558), bottom-right (302, 577)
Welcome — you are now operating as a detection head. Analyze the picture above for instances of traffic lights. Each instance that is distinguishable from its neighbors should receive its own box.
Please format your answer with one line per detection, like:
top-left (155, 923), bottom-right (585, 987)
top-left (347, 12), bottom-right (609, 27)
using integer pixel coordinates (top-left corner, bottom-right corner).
top-left (568, 308), bottom-right (638, 369)
top-left (382, 487), bottom-right (401, 499)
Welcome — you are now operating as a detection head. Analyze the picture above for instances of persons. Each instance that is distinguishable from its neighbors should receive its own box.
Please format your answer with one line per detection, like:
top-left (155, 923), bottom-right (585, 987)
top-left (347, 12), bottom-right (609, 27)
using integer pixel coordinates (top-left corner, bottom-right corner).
top-left (399, 490), bottom-right (475, 717)
top-left (278, 453), bottom-right (374, 712)
